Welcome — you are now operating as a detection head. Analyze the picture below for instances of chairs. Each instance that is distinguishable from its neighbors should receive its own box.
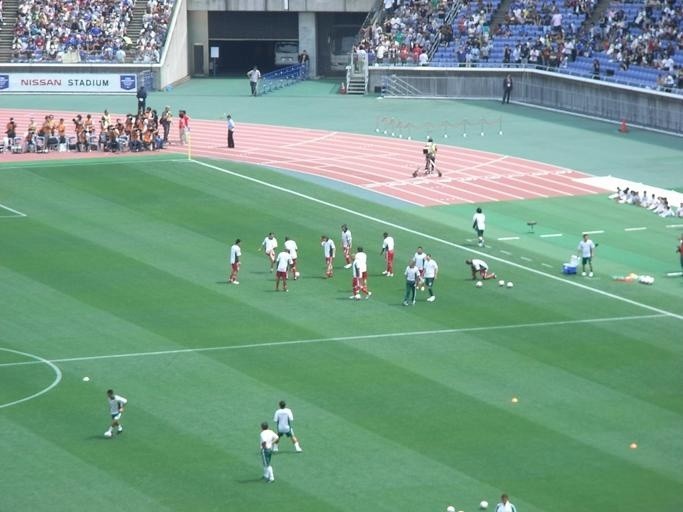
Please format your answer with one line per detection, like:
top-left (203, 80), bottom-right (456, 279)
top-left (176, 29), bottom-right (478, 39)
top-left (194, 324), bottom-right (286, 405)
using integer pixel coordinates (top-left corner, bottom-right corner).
top-left (0, 130), bottom-right (130, 154)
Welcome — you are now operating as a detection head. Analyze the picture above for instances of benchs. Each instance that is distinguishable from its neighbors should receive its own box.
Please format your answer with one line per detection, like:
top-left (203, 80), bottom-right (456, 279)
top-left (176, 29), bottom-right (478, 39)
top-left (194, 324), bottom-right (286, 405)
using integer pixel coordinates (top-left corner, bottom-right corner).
top-left (0, 1), bottom-right (176, 64)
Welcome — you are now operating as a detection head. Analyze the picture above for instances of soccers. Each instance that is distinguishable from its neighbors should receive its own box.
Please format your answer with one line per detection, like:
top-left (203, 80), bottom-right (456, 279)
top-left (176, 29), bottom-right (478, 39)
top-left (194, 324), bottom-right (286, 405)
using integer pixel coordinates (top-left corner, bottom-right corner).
top-left (506, 282), bottom-right (513, 288)
top-left (446, 504), bottom-right (454, 511)
top-left (498, 280), bottom-right (503, 285)
top-left (294, 271), bottom-right (299, 277)
top-left (479, 500), bottom-right (486, 507)
top-left (614, 273), bottom-right (653, 285)
top-left (82, 376), bottom-right (88, 381)
top-left (476, 281), bottom-right (481, 287)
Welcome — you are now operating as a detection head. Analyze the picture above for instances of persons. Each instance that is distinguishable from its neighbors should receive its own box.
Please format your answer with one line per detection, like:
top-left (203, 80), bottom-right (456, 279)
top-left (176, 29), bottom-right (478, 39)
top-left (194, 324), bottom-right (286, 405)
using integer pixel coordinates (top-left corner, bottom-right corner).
top-left (608, 187), bottom-right (683, 218)
top-left (359, 0), bottom-right (682, 93)
top-left (136, 87), bottom-right (147, 114)
top-left (298, 50), bottom-right (309, 62)
top-left (472, 208), bottom-right (485, 247)
top-left (501, 72), bottom-right (513, 105)
top-left (226, 222), bottom-right (438, 306)
top-left (577, 234), bottom-right (599, 277)
top-left (496, 494), bottom-right (516, 512)
top-left (227, 115), bottom-right (236, 149)
top-left (260, 422), bottom-right (279, 482)
top-left (103, 390), bottom-right (128, 437)
top-left (466, 259), bottom-right (497, 280)
top-left (247, 66), bottom-right (261, 96)
top-left (425, 138), bottom-right (437, 173)
top-left (5, 106), bottom-right (191, 152)
top-left (273, 401), bottom-right (302, 453)
top-left (0, 1), bottom-right (176, 64)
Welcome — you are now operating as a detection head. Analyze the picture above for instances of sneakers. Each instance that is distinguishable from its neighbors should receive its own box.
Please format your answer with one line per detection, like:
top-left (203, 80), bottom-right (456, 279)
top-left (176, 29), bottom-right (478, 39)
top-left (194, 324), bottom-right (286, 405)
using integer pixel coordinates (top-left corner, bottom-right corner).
top-left (260, 448), bottom-right (303, 485)
top-left (228, 268), bottom-right (296, 291)
top-left (118, 425), bottom-right (122, 432)
top-left (325, 264), bottom-right (436, 306)
